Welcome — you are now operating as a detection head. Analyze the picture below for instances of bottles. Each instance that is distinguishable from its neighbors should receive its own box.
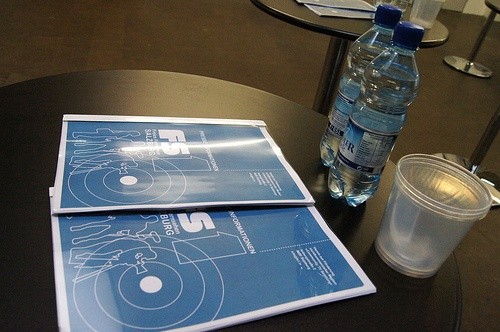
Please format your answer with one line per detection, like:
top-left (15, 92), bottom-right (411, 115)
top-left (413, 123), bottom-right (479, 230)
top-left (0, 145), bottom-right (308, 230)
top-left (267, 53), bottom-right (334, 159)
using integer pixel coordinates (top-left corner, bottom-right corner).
top-left (320, 4), bottom-right (403, 167)
top-left (328, 22), bottom-right (425, 207)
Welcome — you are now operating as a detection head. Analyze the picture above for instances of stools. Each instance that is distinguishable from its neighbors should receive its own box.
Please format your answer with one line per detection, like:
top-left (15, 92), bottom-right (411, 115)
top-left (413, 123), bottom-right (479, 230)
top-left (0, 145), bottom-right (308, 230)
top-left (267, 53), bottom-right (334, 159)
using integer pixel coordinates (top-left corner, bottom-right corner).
top-left (444, 0), bottom-right (500, 78)
top-left (428, 108), bottom-right (500, 212)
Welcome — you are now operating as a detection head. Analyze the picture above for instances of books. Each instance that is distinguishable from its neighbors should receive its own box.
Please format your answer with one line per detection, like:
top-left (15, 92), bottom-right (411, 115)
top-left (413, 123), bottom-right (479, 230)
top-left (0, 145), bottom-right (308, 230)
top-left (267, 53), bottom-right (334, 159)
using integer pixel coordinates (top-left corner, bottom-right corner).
top-left (48, 184), bottom-right (377, 332)
top-left (304, 4), bottom-right (375, 19)
top-left (296, 0), bottom-right (377, 13)
top-left (51, 114), bottom-right (316, 216)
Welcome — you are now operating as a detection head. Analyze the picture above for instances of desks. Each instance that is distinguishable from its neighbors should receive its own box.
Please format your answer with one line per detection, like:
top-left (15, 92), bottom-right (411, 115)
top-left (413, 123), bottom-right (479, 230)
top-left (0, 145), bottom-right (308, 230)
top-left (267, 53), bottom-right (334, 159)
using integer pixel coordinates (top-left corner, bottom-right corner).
top-left (255, 0), bottom-right (449, 116)
top-left (1, 69), bottom-right (463, 331)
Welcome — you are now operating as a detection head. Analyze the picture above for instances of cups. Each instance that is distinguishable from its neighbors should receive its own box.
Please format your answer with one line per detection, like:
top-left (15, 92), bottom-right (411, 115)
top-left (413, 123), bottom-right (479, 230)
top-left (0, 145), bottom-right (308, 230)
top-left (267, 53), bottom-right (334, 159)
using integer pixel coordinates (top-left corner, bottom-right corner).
top-left (374, 152), bottom-right (493, 279)
top-left (408, 0), bottom-right (446, 29)
